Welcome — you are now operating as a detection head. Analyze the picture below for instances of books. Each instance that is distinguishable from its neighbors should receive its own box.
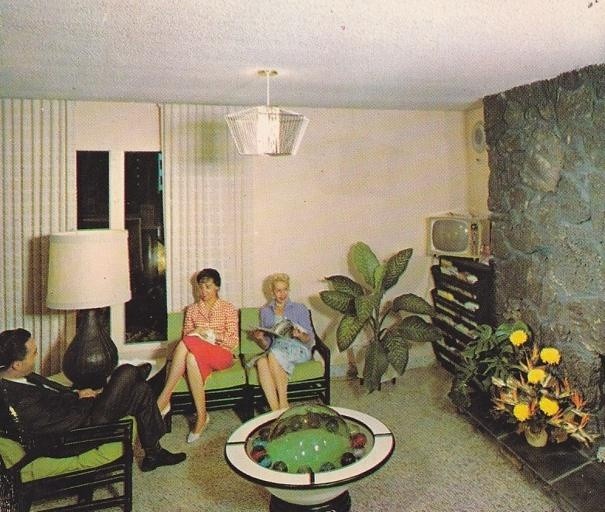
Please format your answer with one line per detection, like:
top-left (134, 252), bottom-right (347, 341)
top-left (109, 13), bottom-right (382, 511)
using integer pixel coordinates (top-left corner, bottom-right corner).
top-left (251, 319), bottom-right (309, 339)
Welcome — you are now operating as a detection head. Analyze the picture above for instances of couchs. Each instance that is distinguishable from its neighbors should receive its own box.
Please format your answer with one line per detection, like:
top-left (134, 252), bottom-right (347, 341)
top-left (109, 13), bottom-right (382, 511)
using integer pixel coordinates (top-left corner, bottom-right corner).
top-left (160, 307), bottom-right (331, 434)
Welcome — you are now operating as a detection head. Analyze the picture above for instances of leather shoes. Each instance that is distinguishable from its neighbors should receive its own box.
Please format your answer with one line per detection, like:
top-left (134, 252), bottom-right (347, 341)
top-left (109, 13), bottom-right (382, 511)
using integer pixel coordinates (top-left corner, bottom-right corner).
top-left (141, 449), bottom-right (186, 471)
top-left (137, 363), bottom-right (151, 380)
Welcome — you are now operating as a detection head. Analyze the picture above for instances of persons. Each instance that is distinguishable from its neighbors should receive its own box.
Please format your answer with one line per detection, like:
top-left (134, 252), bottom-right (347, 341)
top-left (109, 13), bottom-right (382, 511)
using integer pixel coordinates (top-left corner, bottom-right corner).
top-left (0, 327), bottom-right (187, 471)
top-left (156, 267), bottom-right (240, 443)
top-left (251, 272), bottom-right (316, 411)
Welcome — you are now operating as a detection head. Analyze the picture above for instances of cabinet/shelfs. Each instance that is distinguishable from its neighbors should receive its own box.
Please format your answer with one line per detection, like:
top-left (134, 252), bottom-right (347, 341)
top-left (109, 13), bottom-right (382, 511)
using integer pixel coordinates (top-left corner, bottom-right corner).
top-left (430, 257), bottom-right (492, 389)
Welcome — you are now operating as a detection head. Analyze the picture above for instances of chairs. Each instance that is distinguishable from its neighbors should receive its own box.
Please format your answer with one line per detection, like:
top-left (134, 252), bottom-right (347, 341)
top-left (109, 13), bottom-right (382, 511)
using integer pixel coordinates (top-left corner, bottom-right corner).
top-left (0, 385), bottom-right (137, 511)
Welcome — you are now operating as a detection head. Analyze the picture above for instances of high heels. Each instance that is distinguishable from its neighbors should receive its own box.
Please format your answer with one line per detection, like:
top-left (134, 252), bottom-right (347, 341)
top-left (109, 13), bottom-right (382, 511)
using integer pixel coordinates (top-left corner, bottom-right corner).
top-left (187, 412), bottom-right (209, 444)
top-left (161, 402), bottom-right (171, 417)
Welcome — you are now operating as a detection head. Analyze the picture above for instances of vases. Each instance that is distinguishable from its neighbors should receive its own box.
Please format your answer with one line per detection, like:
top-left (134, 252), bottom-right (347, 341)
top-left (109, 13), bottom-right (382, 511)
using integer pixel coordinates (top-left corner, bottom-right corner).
top-left (525, 425), bottom-right (548, 448)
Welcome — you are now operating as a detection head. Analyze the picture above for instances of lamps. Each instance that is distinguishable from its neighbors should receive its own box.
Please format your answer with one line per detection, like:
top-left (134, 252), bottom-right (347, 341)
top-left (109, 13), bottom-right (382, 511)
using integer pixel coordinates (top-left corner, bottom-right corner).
top-left (224, 69), bottom-right (311, 158)
top-left (46, 228), bottom-right (132, 389)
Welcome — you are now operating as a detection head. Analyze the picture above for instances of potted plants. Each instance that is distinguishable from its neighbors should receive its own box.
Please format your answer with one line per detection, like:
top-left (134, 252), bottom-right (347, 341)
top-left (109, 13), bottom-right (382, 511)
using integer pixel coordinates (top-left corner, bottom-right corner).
top-left (318, 241), bottom-right (444, 396)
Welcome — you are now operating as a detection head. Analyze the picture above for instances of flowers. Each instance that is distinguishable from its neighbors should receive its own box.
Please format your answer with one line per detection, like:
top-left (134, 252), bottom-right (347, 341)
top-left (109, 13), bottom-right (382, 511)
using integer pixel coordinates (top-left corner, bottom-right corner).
top-left (492, 328), bottom-right (597, 448)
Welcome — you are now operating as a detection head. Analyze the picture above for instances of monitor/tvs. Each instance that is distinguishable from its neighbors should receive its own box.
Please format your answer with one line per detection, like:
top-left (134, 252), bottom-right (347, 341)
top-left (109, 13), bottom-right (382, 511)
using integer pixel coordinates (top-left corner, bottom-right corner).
top-left (430, 218), bottom-right (490, 259)
